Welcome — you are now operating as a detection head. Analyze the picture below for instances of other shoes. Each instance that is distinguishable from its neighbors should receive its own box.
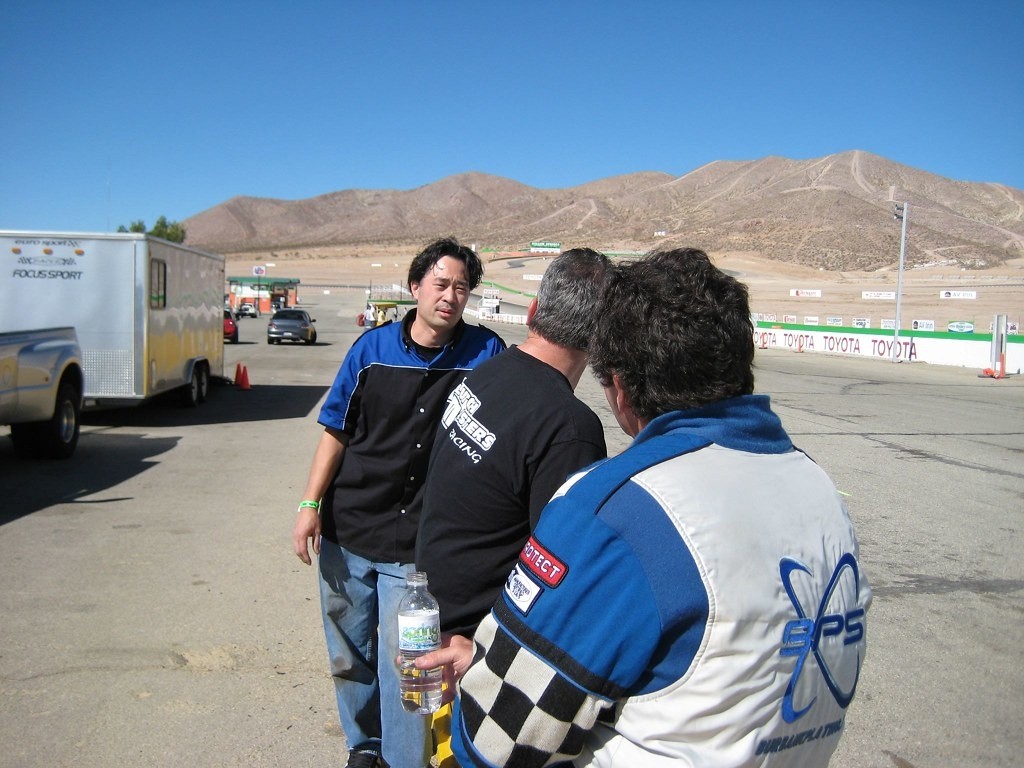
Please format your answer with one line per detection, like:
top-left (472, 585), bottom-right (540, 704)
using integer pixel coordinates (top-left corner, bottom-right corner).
top-left (345, 751), bottom-right (381, 768)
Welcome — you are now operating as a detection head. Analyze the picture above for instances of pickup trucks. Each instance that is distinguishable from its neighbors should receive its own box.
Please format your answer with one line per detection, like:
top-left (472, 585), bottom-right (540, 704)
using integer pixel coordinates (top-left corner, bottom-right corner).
top-left (0, 324), bottom-right (86, 462)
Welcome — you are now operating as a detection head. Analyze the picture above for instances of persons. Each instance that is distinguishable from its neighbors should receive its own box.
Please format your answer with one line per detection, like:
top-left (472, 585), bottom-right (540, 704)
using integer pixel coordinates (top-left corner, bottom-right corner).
top-left (396, 244), bottom-right (872, 768)
top-left (363, 303), bottom-right (408, 329)
top-left (415, 247), bottom-right (618, 648)
top-left (295, 234), bottom-right (508, 768)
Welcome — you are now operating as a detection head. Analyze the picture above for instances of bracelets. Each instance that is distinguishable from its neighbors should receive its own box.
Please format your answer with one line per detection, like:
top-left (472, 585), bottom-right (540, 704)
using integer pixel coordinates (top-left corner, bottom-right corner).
top-left (298, 500), bottom-right (319, 512)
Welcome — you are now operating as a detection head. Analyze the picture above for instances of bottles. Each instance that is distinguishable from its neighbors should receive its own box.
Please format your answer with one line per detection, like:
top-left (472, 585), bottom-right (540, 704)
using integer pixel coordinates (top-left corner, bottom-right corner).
top-left (399, 572), bottom-right (442, 713)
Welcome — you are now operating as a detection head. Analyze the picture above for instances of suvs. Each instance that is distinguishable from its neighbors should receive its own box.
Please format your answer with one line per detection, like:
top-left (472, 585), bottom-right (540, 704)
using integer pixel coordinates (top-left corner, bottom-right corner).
top-left (223, 308), bottom-right (238, 344)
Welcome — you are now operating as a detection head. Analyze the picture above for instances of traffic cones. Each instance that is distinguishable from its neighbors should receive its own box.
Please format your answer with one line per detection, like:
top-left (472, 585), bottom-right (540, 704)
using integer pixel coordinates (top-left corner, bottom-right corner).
top-left (230, 361), bottom-right (243, 387)
top-left (238, 366), bottom-right (255, 391)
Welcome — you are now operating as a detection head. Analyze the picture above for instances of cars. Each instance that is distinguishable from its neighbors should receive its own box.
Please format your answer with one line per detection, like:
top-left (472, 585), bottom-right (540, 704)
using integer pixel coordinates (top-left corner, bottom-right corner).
top-left (232, 303), bottom-right (258, 319)
top-left (267, 308), bottom-right (317, 346)
